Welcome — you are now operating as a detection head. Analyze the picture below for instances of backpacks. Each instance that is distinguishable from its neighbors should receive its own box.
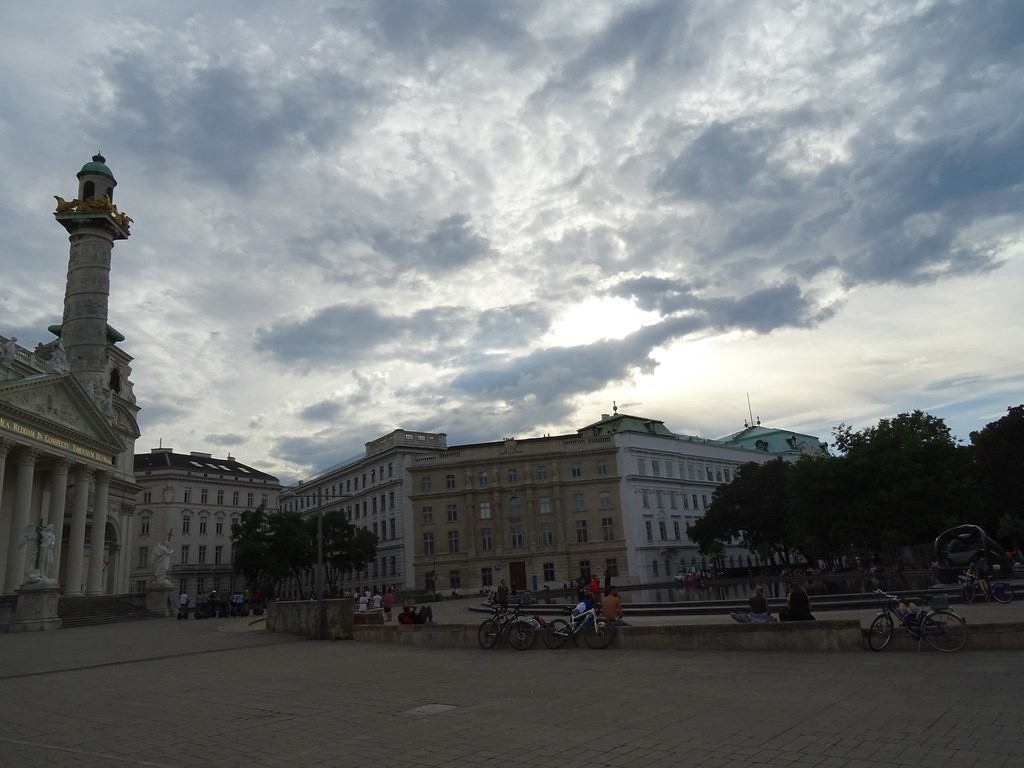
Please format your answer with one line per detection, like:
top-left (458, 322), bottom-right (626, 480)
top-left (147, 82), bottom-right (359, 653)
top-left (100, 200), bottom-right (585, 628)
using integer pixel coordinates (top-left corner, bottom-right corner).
top-left (398, 612), bottom-right (415, 624)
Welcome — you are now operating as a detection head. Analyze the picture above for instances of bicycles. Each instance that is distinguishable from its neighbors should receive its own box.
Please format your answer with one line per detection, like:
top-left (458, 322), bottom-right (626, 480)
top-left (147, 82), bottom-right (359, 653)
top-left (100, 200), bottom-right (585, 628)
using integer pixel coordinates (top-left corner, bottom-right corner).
top-left (478, 596), bottom-right (537, 650)
top-left (958, 568), bottom-right (1015, 604)
top-left (867, 588), bottom-right (970, 653)
top-left (543, 593), bottom-right (618, 650)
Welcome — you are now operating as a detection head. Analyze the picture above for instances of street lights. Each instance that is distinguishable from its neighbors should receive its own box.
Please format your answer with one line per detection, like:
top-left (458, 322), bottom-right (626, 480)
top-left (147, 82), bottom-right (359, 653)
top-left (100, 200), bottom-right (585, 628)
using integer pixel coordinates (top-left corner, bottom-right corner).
top-left (283, 484), bottom-right (356, 639)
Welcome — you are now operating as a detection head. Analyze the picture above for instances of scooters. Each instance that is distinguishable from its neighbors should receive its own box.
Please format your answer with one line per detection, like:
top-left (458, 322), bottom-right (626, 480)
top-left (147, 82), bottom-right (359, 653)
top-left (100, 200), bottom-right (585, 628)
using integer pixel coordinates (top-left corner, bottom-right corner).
top-left (177, 603), bottom-right (189, 620)
top-left (193, 601), bottom-right (264, 618)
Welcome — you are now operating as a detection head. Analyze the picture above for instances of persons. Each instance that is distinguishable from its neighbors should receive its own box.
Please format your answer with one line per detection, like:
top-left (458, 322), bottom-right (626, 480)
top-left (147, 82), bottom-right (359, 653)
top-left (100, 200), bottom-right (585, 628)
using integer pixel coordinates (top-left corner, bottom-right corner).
top-left (450, 588), bottom-right (460, 597)
top-left (749, 585), bottom-right (770, 623)
top-left (151, 539), bottom-right (175, 583)
top-left (354, 588), bottom-right (434, 625)
top-left (782, 555), bottom-right (847, 576)
top-left (675, 571), bottom-right (712, 580)
top-left (966, 562), bottom-right (989, 594)
top-left (778, 582), bottom-right (815, 621)
top-left (479, 566), bottom-right (623, 625)
top-left (899, 603), bottom-right (946, 626)
top-left (180, 587), bottom-right (258, 614)
top-left (17, 523), bottom-right (57, 583)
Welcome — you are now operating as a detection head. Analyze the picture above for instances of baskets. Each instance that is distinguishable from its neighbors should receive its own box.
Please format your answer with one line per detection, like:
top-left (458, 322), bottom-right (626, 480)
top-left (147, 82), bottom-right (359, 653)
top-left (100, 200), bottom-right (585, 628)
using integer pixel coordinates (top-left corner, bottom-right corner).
top-left (930, 597), bottom-right (950, 611)
